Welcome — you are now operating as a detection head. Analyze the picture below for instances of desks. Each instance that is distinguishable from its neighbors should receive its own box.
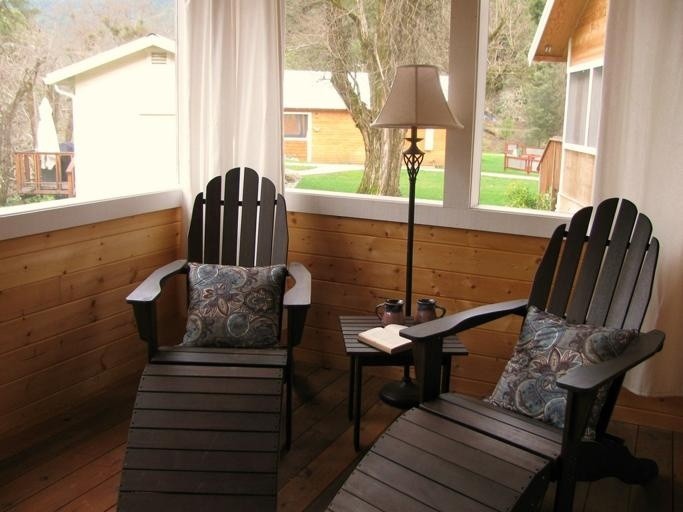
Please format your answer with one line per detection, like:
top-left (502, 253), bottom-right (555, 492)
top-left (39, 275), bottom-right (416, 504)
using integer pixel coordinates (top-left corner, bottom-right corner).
top-left (337, 311), bottom-right (469, 450)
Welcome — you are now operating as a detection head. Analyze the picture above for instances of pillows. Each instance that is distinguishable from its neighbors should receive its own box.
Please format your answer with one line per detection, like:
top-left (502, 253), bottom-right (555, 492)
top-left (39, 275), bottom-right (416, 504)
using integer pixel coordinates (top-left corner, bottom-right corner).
top-left (484, 302), bottom-right (637, 449)
top-left (181, 260), bottom-right (288, 348)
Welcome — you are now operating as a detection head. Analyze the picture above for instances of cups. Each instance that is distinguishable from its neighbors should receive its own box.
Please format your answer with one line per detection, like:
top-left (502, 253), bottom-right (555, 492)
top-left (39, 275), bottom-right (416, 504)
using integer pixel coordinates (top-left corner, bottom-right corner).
top-left (374, 299), bottom-right (407, 329)
top-left (415, 297), bottom-right (446, 326)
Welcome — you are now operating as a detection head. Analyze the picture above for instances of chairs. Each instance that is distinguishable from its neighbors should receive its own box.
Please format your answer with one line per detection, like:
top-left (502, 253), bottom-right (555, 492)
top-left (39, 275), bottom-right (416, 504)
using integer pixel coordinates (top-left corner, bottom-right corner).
top-left (328, 193), bottom-right (669, 511)
top-left (113, 163), bottom-right (314, 512)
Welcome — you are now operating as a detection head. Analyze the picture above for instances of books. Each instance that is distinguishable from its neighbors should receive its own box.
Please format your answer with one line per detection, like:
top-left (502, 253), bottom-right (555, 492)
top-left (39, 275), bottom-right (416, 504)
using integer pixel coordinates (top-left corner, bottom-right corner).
top-left (357, 323), bottom-right (424, 354)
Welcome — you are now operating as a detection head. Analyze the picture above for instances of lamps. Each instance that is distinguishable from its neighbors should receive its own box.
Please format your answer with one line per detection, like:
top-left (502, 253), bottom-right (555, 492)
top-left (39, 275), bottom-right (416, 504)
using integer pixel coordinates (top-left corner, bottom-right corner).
top-left (368, 63), bottom-right (467, 415)
